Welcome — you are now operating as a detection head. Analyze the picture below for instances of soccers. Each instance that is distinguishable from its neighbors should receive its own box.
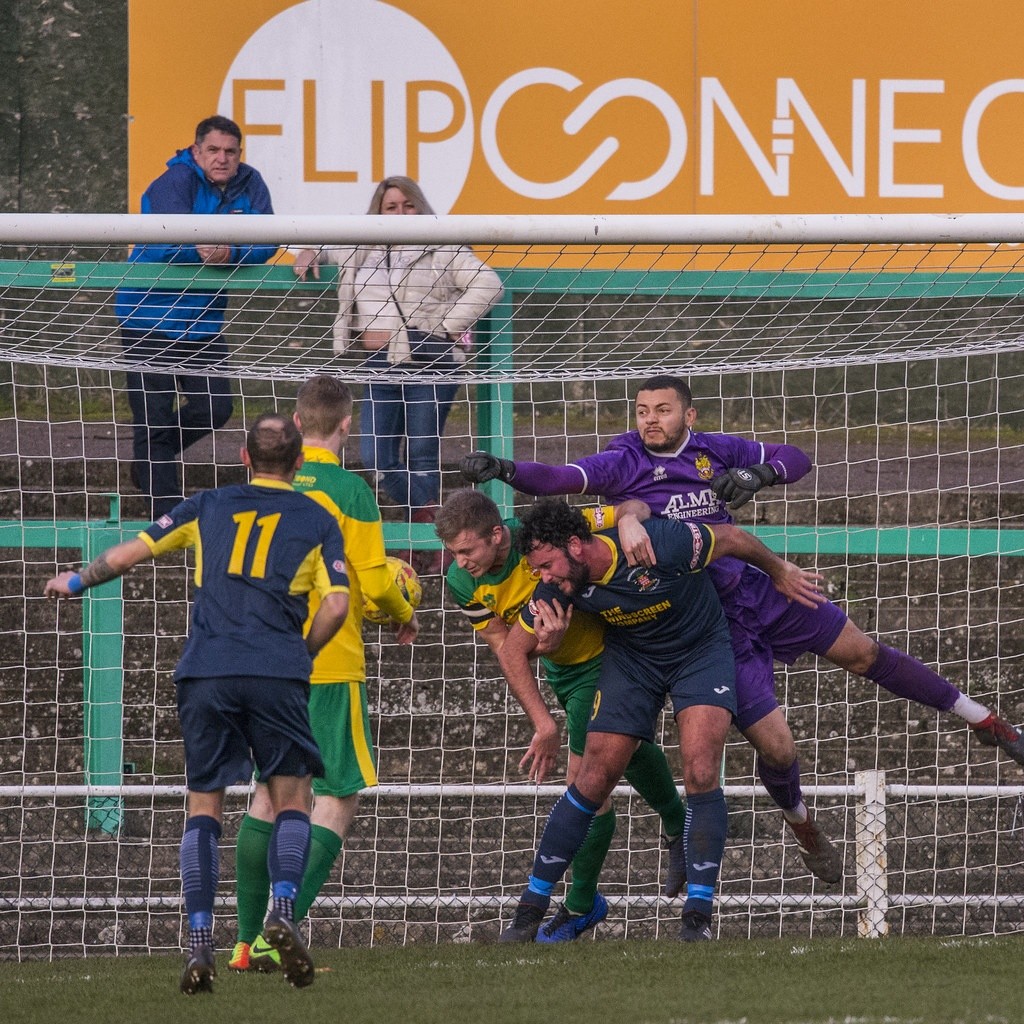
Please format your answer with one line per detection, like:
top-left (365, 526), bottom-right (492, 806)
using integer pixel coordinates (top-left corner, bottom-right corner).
top-left (361, 556), bottom-right (423, 624)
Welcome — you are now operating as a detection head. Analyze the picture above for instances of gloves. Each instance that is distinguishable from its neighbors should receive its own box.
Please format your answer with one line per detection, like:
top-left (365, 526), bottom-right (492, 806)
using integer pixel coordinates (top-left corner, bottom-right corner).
top-left (709, 463), bottom-right (774, 511)
top-left (458, 452), bottom-right (514, 483)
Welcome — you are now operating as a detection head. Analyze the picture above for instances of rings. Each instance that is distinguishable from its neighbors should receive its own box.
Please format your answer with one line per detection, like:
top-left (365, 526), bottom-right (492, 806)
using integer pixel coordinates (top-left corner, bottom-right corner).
top-left (296, 267), bottom-right (302, 270)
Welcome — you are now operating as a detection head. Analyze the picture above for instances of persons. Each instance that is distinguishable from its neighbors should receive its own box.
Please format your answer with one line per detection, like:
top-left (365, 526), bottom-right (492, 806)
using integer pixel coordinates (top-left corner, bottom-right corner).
top-left (434, 374), bottom-right (1024, 943)
top-left (45, 375), bottom-right (420, 994)
top-left (293, 176), bottom-right (505, 574)
top-left (112, 114), bottom-right (280, 522)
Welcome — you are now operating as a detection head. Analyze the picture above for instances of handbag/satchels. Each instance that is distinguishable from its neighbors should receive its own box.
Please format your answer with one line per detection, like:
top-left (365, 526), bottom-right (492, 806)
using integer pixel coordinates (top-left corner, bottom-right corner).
top-left (407, 328), bottom-right (454, 365)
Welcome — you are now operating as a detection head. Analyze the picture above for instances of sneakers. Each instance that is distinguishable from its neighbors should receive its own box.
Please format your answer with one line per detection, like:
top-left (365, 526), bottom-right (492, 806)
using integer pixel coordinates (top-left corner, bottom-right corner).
top-left (179, 945), bottom-right (218, 995)
top-left (228, 934), bottom-right (282, 972)
top-left (678, 897), bottom-right (713, 943)
top-left (966, 710), bottom-right (1024, 766)
top-left (534, 890), bottom-right (609, 943)
top-left (497, 888), bottom-right (551, 943)
top-left (660, 834), bottom-right (687, 899)
top-left (781, 799), bottom-right (843, 884)
top-left (264, 910), bottom-right (316, 989)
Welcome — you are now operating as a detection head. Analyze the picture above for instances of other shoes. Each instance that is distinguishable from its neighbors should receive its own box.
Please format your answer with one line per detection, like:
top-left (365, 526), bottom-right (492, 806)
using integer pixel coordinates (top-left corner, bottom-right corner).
top-left (129, 459), bottom-right (141, 490)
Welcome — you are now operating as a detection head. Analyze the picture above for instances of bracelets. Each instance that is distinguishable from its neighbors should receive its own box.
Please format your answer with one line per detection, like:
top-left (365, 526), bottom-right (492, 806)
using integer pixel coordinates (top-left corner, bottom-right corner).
top-left (67, 573), bottom-right (85, 593)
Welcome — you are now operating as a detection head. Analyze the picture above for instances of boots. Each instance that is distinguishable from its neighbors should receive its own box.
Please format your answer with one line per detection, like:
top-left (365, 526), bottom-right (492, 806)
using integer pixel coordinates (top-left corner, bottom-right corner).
top-left (398, 499), bottom-right (454, 574)
